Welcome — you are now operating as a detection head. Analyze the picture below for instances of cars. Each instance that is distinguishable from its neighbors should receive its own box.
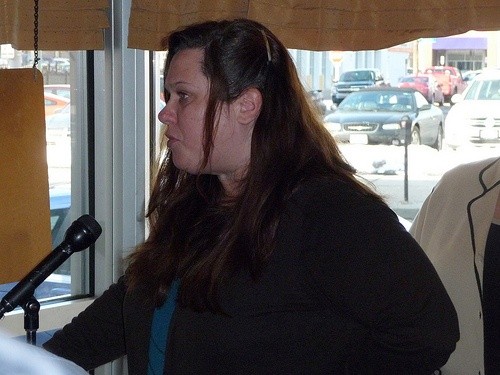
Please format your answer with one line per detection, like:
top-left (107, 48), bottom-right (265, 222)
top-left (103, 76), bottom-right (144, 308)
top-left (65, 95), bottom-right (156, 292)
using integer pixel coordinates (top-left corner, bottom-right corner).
top-left (444, 75), bottom-right (500, 149)
top-left (323, 87), bottom-right (444, 152)
top-left (400, 65), bottom-right (466, 106)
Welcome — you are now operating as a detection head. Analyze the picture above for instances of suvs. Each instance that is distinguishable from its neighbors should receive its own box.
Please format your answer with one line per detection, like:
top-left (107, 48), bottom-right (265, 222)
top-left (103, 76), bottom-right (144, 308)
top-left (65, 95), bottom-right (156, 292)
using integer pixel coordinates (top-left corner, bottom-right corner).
top-left (333, 70), bottom-right (385, 104)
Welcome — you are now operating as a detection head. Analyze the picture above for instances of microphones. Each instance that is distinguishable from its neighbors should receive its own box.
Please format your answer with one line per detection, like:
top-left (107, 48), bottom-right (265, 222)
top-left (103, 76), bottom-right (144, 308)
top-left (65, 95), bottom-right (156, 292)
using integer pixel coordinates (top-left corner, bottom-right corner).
top-left (0, 214), bottom-right (102, 319)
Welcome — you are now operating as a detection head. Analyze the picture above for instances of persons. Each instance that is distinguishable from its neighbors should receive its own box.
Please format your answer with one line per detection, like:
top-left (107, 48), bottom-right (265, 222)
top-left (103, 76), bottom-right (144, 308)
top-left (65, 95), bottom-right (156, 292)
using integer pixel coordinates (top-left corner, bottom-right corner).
top-left (407, 157), bottom-right (500, 375)
top-left (40, 19), bottom-right (460, 375)
